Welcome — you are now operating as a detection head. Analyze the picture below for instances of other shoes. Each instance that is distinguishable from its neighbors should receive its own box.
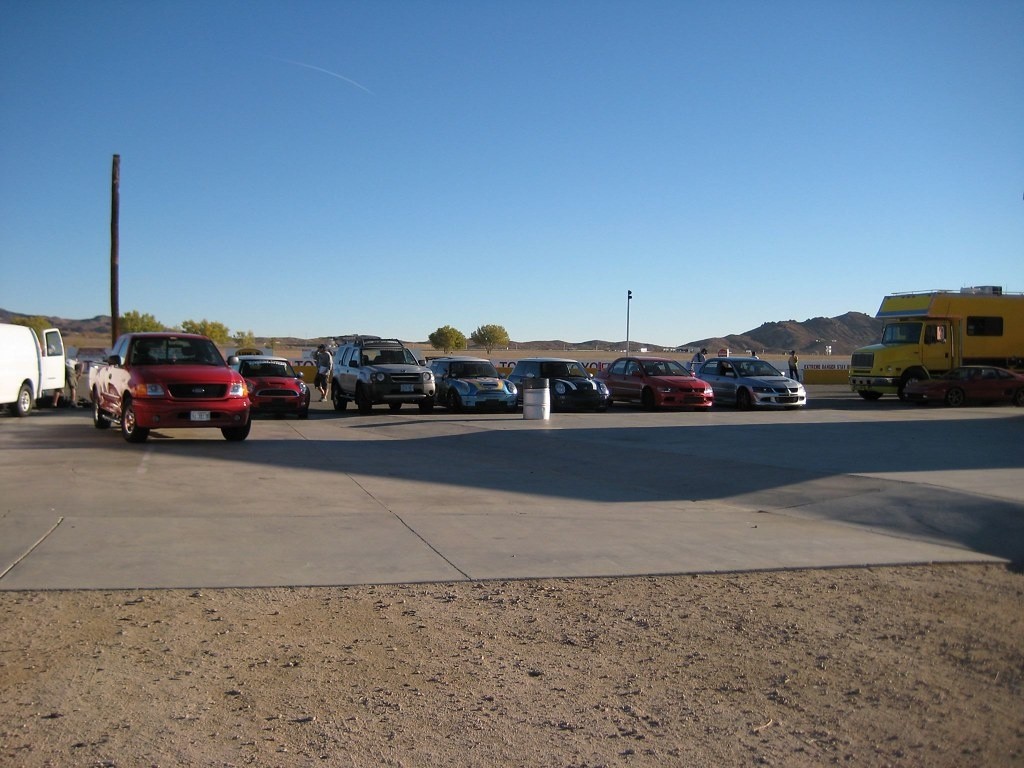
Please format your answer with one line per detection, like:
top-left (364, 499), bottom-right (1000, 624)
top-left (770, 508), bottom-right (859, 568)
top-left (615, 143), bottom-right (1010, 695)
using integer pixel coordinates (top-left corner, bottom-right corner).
top-left (319, 397), bottom-right (327, 402)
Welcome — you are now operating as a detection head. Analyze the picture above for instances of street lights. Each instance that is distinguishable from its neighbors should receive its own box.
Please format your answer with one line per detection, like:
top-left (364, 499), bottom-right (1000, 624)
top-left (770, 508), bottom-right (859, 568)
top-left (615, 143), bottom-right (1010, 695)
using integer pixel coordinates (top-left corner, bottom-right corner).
top-left (626, 289), bottom-right (632, 356)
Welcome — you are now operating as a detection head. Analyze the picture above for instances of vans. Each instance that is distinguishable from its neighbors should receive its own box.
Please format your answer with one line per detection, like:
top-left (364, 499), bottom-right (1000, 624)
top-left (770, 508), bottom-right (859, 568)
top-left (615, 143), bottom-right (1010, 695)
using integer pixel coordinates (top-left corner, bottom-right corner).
top-left (0, 320), bottom-right (65, 417)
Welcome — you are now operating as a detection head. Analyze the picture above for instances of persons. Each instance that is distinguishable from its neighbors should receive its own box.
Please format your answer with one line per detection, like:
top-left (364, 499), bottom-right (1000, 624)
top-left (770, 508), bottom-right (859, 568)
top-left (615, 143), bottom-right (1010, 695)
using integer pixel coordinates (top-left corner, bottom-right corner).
top-left (690, 348), bottom-right (708, 377)
top-left (750, 351), bottom-right (759, 359)
top-left (788, 350), bottom-right (800, 382)
top-left (51, 359), bottom-right (83, 410)
top-left (313, 344), bottom-right (332, 402)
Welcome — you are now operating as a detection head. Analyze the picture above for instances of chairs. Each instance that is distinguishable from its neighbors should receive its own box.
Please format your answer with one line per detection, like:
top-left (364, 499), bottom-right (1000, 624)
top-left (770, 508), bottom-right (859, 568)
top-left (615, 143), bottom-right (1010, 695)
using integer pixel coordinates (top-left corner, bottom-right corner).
top-left (174, 347), bottom-right (199, 363)
top-left (467, 367), bottom-right (476, 375)
top-left (362, 355), bottom-right (370, 365)
top-left (628, 366), bottom-right (639, 375)
top-left (133, 343), bottom-right (157, 364)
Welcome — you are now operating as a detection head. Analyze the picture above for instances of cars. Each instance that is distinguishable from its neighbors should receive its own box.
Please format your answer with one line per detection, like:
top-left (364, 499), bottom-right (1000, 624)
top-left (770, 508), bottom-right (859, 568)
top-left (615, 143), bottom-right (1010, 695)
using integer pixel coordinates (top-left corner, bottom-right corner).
top-left (422, 356), bottom-right (517, 415)
top-left (902, 365), bottom-right (1024, 408)
top-left (506, 358), bottom-right (611, 413)
top-left (593, 356), bottom-right (714, 412)
top-left (686, 356), bottom-right (806, 411)
top-left (230, 353), bottom-right (311, 419)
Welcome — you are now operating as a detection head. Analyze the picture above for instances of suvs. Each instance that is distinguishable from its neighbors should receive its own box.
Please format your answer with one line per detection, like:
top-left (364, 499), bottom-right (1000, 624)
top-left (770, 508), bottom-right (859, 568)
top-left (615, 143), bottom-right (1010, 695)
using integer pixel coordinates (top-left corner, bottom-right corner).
top-left (330, 337), bottom-right (436, 416)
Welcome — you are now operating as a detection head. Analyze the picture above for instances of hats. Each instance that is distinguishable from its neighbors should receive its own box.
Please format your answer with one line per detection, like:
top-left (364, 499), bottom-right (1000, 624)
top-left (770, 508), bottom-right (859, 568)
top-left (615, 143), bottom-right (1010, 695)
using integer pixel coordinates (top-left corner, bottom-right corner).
top-left (703, 348), bottom-right (708, 354)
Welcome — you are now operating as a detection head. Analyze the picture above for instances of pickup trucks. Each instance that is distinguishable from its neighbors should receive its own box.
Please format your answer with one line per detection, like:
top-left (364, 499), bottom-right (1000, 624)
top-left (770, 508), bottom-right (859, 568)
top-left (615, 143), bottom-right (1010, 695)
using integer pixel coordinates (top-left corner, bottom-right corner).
top-left (86, 332), bottom-right (253, 445)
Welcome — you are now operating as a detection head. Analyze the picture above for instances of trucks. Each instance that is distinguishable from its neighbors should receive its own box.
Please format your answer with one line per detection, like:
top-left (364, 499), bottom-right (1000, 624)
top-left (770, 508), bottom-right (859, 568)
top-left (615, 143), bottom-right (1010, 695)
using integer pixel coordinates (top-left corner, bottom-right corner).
top-left (849, 286), bottom-right (1024, 403)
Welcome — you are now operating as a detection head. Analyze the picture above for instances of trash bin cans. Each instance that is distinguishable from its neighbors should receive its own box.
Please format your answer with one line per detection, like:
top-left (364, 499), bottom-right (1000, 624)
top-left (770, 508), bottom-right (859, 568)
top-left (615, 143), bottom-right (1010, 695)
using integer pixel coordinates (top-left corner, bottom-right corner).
top-left (523, 377), bottom-right (550, 420)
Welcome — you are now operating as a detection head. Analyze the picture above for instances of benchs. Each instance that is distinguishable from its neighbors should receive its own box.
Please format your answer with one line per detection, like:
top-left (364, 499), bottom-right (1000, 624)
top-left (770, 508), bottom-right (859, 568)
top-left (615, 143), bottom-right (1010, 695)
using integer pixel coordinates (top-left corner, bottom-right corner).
top-left (614, 367), bottom-right (624, 374)
